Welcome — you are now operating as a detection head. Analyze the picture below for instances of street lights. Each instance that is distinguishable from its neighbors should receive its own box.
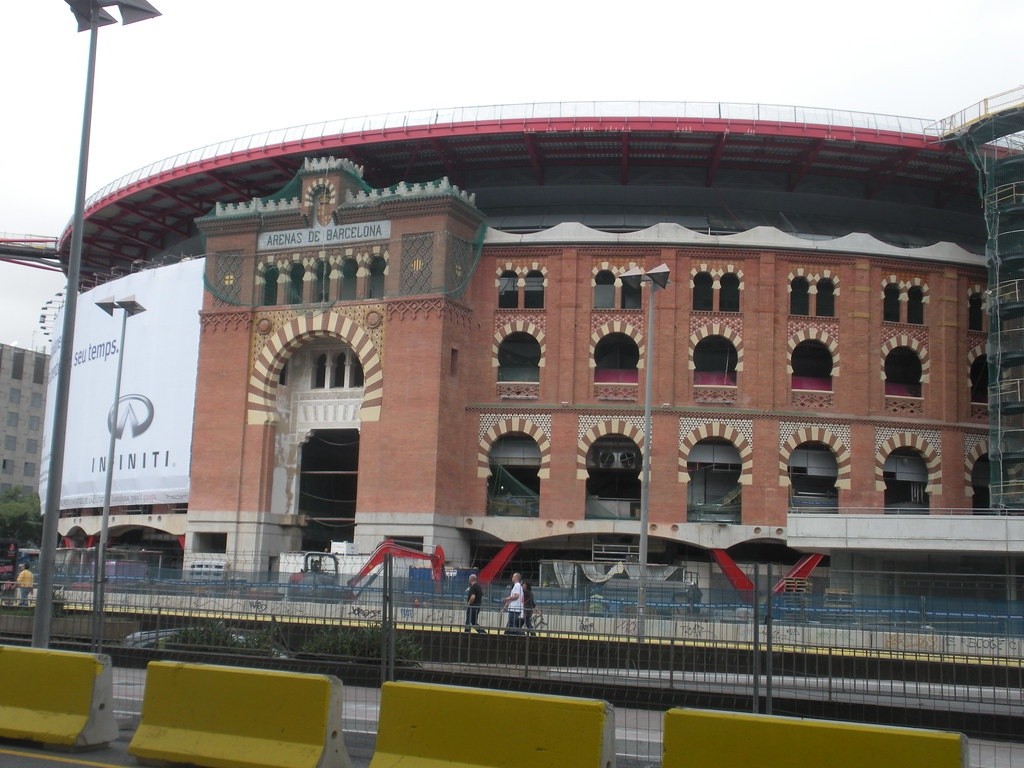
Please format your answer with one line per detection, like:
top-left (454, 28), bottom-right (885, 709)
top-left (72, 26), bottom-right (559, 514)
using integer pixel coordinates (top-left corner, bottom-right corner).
top-left (95, 292), bottom-right (146, 655)
top-left (617, 263), bottom-right (670, 644)
top-left (28, 1), bottom-right (163, 654)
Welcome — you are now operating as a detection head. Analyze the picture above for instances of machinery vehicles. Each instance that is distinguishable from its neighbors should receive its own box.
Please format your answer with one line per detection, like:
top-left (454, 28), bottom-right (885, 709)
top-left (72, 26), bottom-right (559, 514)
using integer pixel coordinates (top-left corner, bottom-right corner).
top-left (288, 541), bottom-right (448, 604)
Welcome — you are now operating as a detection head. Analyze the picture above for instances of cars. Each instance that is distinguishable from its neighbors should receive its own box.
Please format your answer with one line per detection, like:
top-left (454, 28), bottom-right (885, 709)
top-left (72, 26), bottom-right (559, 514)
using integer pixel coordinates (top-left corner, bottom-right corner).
top-left (126, 626), bottom-right (298, 658)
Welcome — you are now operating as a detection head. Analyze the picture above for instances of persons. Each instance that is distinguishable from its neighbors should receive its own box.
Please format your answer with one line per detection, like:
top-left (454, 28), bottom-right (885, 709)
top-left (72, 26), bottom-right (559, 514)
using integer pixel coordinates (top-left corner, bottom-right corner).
top-left (518, 583), bottom-right (536, 636)
top-left (11, 563), bottom-right (34, 606)
top-left (504, 573), bottom-right (524, 634)
top-left (465, 573), bottom-right (487, 633)
